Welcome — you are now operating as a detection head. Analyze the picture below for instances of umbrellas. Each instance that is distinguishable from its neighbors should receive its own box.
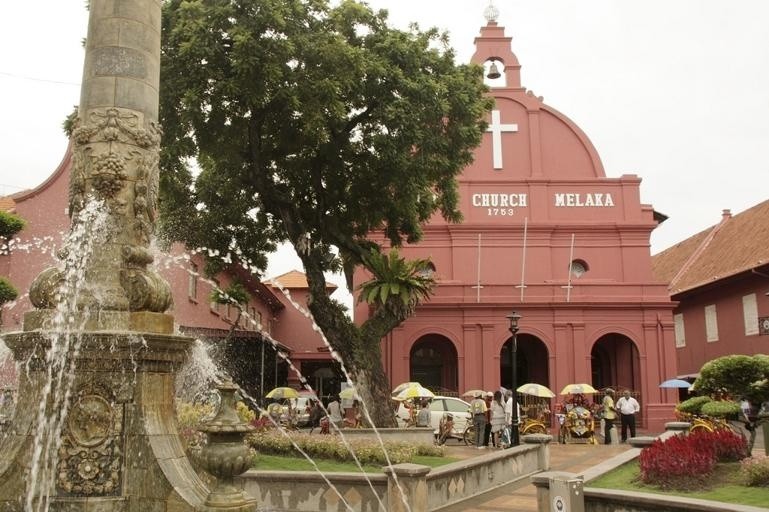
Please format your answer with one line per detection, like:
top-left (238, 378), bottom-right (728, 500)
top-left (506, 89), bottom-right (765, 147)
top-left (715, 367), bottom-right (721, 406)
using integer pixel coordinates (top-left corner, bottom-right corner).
top-left (392, 382), bottom-right (434, 401)
top-left (516, 383), bottom-right (556, 398)
top-left (339, 388), bottom-right (352, 399)
top-left (265, 387), bottom-right (302, 398)
top-left (560, 383), bottom-right (599, 395)
top-left (658, 379), bottom-right (692, 404)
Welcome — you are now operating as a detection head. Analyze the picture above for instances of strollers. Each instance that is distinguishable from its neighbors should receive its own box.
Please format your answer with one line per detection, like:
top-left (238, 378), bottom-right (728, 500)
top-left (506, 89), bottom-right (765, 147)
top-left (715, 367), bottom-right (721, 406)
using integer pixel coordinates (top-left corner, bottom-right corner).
top-left (319, 415), bottom-right (329, 434)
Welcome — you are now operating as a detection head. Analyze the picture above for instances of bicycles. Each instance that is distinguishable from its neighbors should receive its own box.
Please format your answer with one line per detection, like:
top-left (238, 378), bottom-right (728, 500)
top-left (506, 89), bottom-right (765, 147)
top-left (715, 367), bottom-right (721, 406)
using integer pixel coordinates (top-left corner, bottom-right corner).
top-left (676, 412), bottom-right (734, 435)
top-left (519, 415), bottom-right (547, 435)
top-left (437, 412), bottom-right (474, 445)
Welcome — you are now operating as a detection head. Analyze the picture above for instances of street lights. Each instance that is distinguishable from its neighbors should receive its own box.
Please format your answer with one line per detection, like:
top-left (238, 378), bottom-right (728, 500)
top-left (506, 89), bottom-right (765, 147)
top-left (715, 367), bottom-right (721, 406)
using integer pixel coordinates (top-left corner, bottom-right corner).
top-left (506, 311), bottom-right (523, 448)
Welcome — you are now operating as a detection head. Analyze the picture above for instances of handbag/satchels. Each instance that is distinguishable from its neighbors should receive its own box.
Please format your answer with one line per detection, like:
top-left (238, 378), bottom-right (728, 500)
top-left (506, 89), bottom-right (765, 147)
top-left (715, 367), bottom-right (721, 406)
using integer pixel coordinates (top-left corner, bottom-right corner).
top-left (500, 424), bottom-right (512, 446)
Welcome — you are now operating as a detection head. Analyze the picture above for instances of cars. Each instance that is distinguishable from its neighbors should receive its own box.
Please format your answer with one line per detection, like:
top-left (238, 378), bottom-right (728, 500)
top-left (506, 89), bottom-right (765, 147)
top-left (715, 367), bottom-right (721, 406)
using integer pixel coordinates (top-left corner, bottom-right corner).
top-left (280, 397), bottom-right (321, 426)
top-left (394, 396), bottom-right (474, 434)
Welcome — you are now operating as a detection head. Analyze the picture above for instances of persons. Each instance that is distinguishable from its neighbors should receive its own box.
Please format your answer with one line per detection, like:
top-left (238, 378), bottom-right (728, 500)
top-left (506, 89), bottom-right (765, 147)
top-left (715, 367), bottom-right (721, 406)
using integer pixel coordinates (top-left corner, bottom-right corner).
top-left (437, 413), bottom-right (464, 445)
top-left (352, 400), bottom-right (362, 429)
top-left (603, 388), bottom-right (640, 444)
top-left (311, 396), bottom-right (345, 435)
top-left (741, 401), bottom-right (758, 456)
top-left (418, 402), bottom-right (431, 426)
top-left (471, 389), bottom-right (520, 449)
